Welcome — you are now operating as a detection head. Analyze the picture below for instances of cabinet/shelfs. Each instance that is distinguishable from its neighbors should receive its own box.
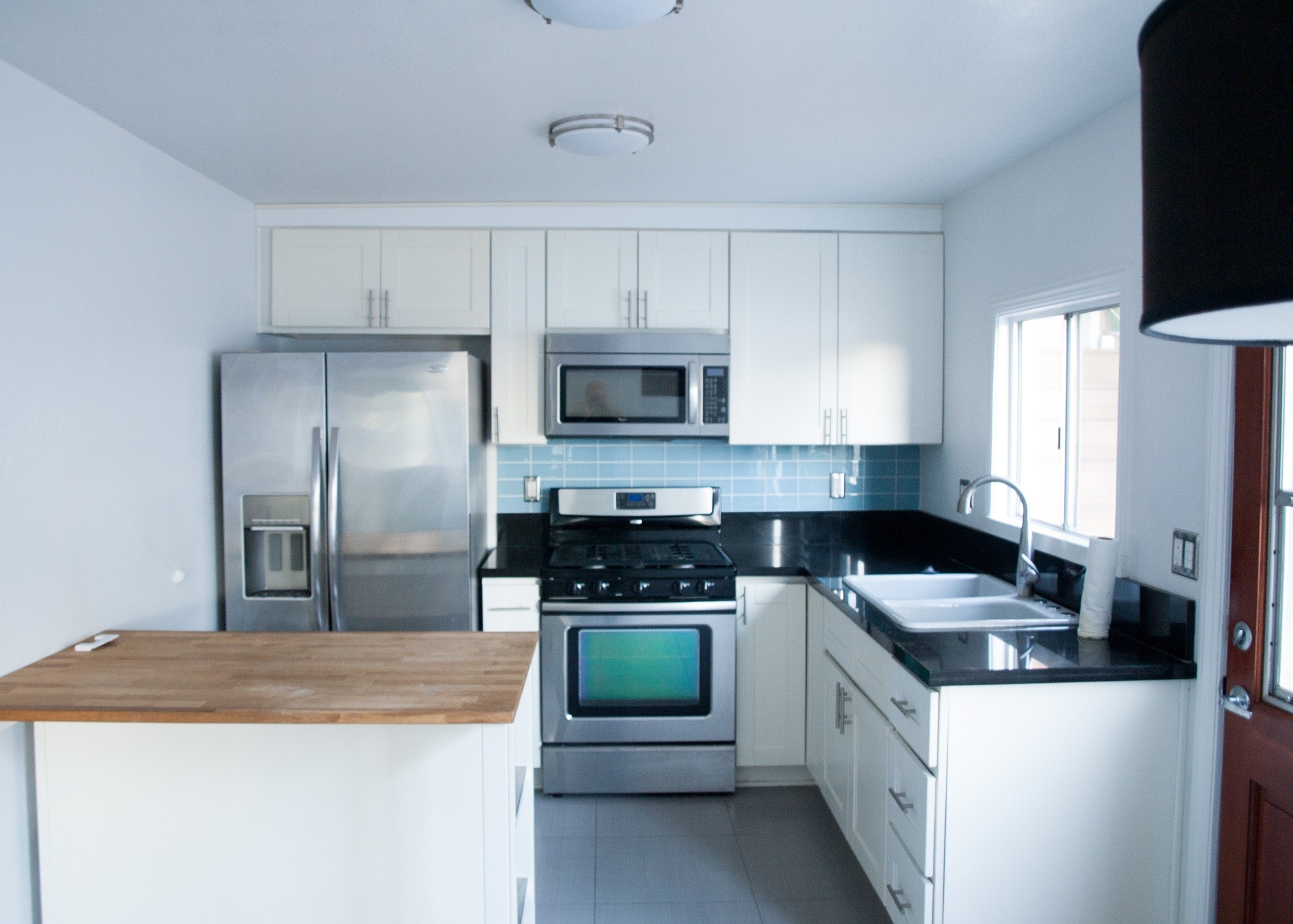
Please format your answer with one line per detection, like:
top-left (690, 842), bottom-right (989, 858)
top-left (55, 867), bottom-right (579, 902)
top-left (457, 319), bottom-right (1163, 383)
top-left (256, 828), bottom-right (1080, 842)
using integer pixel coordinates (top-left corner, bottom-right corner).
top-left (808, 585), bottom-right (1191, 924)
top-left (547, 230), bottom-right (729, 331)
top-left (492, 228), bottom-right (548, 446)
top-left (482, 579), bottom-right (542, 790)
top-left (737, 576), bottom-right (807, 782)
top-left (270, 227), bottom-right (491, 330)
top-left (729, 230), bottom-right (943, 447)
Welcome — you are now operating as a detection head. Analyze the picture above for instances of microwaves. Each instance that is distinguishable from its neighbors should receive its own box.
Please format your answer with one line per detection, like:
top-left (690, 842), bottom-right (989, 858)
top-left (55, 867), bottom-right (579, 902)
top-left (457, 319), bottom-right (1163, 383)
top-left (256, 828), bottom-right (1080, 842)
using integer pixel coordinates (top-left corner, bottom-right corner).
top-left (545, 331), bottom-right (730, 438)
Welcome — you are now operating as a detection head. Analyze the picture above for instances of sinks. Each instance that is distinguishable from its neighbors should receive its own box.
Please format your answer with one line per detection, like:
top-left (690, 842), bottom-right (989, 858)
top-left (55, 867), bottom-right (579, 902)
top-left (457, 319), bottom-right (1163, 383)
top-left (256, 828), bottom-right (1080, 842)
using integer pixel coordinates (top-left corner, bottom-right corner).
top-left (876, 595), bottom-right (1083, 633)
top-left (842, 571), bottom-right (1023, 607)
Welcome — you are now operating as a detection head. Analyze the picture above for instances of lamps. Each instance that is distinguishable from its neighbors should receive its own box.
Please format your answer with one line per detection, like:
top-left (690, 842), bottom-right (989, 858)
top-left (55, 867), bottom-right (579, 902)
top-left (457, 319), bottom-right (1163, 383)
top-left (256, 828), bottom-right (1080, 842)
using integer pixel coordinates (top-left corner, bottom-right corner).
top-left (549, 115), bottom-right (655, 157)
top-left (524, 1), bottom-right (684, 29)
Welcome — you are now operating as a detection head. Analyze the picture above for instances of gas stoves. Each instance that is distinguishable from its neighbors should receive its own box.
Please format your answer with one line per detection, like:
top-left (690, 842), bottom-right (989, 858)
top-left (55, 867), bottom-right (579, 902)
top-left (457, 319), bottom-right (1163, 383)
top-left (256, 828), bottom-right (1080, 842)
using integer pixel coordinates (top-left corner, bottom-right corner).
top-left (545, 486), bottom-right (738, 603)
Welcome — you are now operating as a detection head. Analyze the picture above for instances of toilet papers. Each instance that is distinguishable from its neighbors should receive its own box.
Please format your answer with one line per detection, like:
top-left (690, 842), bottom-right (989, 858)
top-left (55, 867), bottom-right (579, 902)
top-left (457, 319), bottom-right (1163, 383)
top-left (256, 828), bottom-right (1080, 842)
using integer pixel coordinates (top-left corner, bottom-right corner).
top-left (1077, 536), bottom-right (1120, 641)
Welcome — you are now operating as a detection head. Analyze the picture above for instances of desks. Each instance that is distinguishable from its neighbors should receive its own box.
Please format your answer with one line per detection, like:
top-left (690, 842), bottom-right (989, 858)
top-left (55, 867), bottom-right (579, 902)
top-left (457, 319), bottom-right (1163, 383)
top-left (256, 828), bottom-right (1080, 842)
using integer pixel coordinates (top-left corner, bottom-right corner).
top-left (0, 630), bottom-right (541, 924)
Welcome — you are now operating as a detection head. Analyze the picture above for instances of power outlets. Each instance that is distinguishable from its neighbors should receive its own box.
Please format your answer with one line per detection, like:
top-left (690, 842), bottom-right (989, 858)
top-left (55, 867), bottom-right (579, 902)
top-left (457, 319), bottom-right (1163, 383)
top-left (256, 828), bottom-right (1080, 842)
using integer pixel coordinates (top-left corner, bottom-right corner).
top-left (959, 479), bottom-right (972, 514)
top-left (830, 472), bottom-right (846, 499)
top-left (524, 476), bottom-right (540, 502)
top-left (1171, 528), bottom-right (1200, 581)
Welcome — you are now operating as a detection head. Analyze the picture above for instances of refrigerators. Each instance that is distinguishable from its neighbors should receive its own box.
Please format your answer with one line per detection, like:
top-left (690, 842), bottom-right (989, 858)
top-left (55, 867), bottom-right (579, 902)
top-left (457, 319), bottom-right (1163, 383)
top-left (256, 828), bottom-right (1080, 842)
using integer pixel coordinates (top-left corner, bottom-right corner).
top-left (221, 351), bottom-right (487, 634)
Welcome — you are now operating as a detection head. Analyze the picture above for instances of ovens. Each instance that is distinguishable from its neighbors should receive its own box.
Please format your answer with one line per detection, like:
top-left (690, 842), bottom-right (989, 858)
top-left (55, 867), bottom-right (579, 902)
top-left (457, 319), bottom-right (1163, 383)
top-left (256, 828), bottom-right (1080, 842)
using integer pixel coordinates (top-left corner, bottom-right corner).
top-left (540, 602), bottom-right (737, 796)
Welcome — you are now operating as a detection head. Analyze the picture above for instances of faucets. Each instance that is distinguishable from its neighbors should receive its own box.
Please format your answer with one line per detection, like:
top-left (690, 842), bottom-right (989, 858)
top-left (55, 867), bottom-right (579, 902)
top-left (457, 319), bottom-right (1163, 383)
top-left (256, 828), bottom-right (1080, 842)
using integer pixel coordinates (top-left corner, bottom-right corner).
top-left (957, 477), bottom-right (1042, 598)
